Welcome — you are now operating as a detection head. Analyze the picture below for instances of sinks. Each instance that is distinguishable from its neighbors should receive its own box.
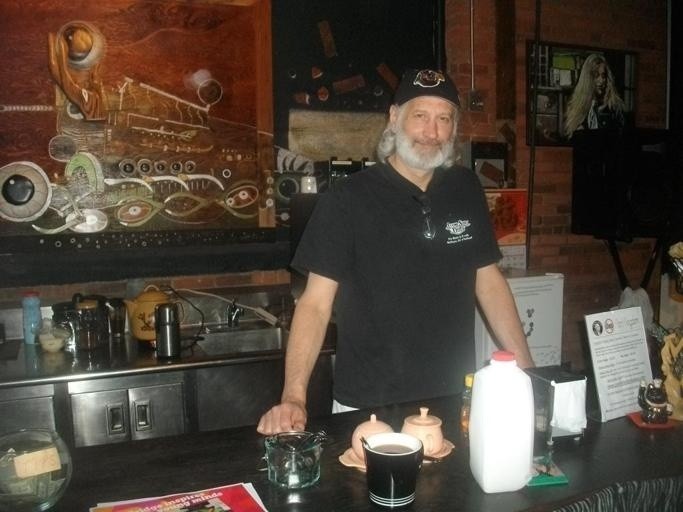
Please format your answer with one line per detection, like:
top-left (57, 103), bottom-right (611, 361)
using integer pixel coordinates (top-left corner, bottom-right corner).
top-left (193, 327), bottom-right (290, 356)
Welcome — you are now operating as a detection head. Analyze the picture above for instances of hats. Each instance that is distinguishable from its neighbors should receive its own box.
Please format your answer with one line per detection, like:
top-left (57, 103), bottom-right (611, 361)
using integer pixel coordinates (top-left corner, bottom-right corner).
top-left (394, 67), bottom-right (461, 109)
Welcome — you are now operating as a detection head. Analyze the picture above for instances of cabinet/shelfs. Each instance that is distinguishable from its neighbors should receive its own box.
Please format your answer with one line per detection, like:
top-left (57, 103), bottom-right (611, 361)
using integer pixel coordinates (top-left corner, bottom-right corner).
top-left (69, 382), bottom-right (185, 448)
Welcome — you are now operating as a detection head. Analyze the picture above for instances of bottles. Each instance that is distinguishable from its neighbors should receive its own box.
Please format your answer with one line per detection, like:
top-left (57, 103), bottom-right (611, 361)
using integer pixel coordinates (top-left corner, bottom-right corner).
top-left (154, 303), bottom-right (182, 358)
top-left (21, 292), bottom-right (44, 346)
top-left (48, 294), bottom-right (127, 351)
top-left (459, 373), bottom-right (474, 437)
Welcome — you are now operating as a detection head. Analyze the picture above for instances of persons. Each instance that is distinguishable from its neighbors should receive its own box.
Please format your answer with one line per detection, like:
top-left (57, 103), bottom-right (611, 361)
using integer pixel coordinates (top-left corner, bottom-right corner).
top-left (594, 323), bottom-right (602, 335)
top-left (543, 52), bottom-right (629, 143)
top-left (255, 65), bottom-right (536, 438)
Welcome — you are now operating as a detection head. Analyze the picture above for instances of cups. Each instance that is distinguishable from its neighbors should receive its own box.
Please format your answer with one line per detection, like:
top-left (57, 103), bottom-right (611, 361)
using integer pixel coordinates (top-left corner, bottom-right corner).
top-left (264, 430), bottom-right (322, 490)
top-left (300, 176), bottom-right (318, 195)
top-left (362, 432), bottom-right (424, 512)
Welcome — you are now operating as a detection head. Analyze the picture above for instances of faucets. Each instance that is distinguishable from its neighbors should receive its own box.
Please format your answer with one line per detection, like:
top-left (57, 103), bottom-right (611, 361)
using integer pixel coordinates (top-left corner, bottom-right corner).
top-left (225, 297), bottom-right (245, 327)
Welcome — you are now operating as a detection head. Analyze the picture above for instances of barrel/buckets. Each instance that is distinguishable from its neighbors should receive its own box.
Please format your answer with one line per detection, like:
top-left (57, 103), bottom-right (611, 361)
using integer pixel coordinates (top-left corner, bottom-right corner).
top-left (465, 353), bottom-right (540, 496)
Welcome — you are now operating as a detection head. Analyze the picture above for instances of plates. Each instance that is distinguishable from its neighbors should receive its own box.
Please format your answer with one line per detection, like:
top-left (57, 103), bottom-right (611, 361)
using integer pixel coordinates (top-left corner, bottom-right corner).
top-left (338, 438), bottom-right (456, 473)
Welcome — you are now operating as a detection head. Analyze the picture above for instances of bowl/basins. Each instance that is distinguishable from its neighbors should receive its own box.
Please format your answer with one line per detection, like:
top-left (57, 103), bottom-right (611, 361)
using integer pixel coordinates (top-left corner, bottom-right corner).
top-left (36, 330), bottom-right (69, 352)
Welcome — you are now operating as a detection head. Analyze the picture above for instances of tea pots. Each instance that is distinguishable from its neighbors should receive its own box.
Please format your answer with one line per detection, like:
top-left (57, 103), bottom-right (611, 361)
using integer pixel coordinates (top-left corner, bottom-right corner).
top-left (352, 406), bottom-right (443, 461)
top-left (124, 285), bottom-right (174, 342)
